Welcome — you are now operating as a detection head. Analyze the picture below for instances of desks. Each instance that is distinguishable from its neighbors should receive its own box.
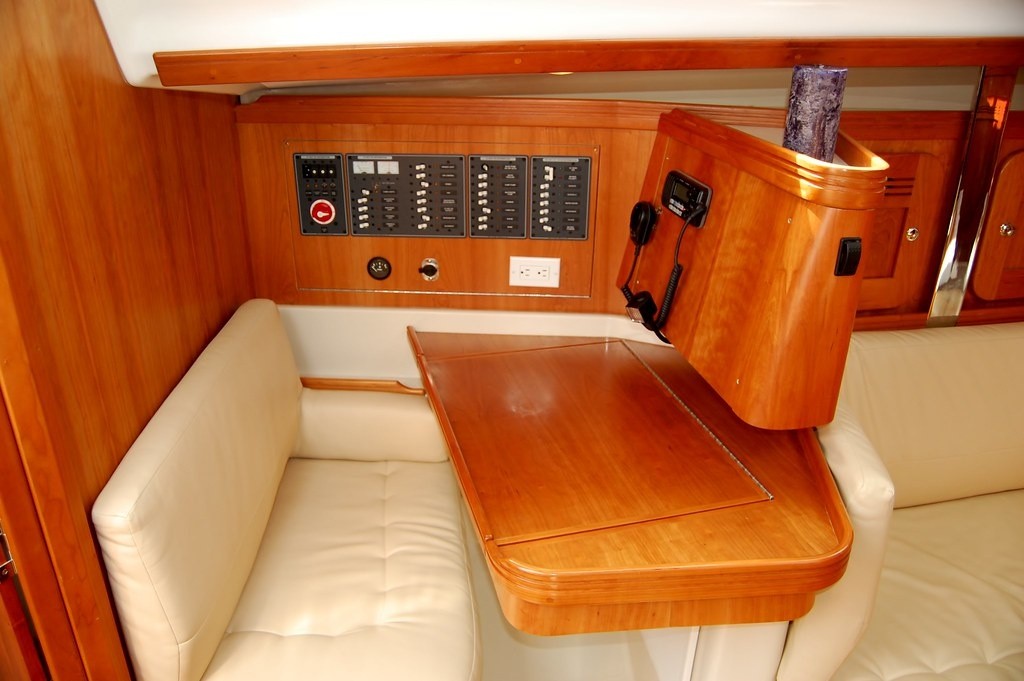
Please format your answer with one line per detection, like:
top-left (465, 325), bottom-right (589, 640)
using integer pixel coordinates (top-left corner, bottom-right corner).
top-left (404, 322), bottom-right (855, 634)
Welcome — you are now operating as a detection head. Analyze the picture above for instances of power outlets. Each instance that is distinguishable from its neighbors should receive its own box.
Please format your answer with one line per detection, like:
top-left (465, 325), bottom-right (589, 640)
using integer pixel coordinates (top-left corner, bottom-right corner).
top-left (508, 256), bottom-right (561, 289)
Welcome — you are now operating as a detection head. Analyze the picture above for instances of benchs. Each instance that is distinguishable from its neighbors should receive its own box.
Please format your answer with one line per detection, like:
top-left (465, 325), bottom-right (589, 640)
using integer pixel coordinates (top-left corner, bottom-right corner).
top-left (777, 316), bottom-right (1024, 681)
top-left (93, 297), bottom-right (484, 681)
